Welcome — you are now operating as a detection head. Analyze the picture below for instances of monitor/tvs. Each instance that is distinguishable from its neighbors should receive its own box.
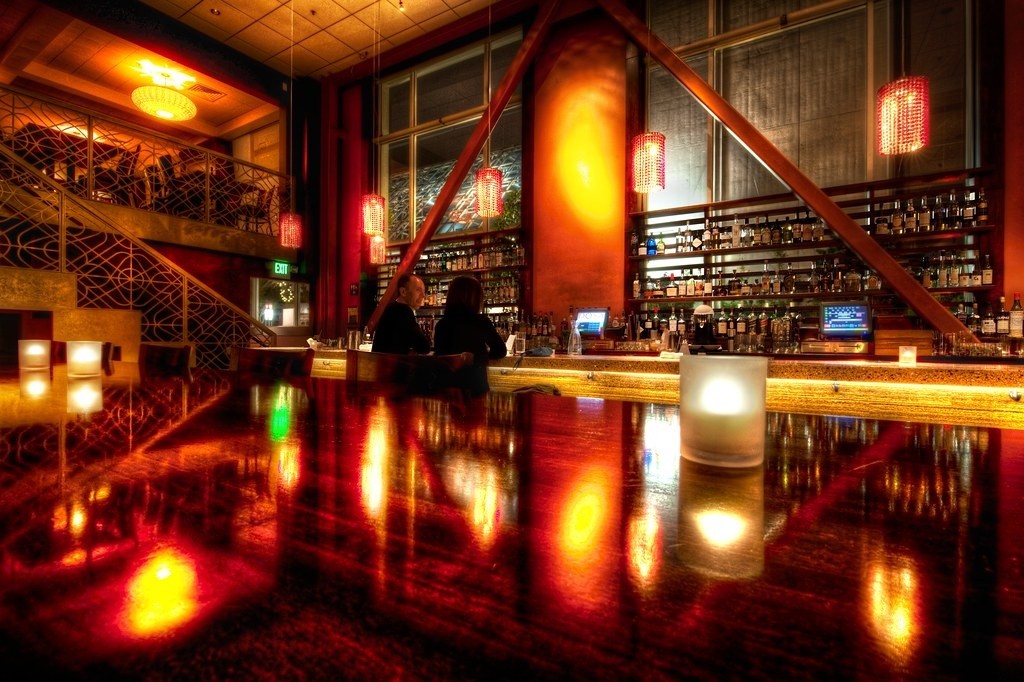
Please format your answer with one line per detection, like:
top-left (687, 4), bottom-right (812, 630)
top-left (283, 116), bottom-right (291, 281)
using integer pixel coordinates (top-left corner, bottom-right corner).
top-left (820, 301), bottom-right (872, 336)
top-left (575, 309), bottom-right (607, 336)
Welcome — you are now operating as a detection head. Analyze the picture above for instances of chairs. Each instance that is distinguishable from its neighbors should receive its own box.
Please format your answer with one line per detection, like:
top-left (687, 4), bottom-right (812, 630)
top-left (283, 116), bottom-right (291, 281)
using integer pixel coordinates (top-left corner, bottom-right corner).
top-left (346, 347), bottom-right (473, 385)
top-left (228, 343), bottom-right (315, 378)
top-left (12, 144), bottom-right (278, 238)
top-left (135, 342), bottom-right (192, 367)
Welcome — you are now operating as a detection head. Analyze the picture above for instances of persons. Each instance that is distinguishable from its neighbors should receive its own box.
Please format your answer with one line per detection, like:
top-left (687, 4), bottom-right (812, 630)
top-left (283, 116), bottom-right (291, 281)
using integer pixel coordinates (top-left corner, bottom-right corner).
top-left (434, 275), bottom-right (507, 380)
top-left (371, 272), bottom-right (432, 354)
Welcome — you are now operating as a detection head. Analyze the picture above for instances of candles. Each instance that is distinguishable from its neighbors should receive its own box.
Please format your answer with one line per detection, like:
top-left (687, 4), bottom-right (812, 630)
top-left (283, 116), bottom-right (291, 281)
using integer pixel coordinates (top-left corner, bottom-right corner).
top-left (676, 463), bottom-right (766, 582)
top-left (68, 347), bottom-right (101, 375)
top-left (21, 344), bottom-right (49, 369)
top-left (66, 376), bottom-right (103, 413)
top-left (20, 369), bottom-right (50, 399)
top-left (681, 377), bottom-right (765, 453)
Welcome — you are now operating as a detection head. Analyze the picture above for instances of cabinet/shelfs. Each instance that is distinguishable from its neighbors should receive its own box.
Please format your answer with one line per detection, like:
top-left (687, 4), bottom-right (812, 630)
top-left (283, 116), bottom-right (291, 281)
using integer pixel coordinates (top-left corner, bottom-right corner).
top-left (630, 167), bottom-right (995, 320)
top-left (372, 223), bottom-right (531, 343)
top-left (328, 383), bottom-right (532, 623)
top-left (763, 412), bottom-right (996, 626)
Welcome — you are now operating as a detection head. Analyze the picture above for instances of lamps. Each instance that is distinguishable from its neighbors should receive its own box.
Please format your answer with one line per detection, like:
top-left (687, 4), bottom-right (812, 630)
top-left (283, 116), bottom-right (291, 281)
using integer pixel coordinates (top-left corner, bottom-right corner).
top-left (629, 0), bottom-right (669, 194)
top-left (360, 0), bottom-right (385, 236)
top-left (367, 0), bottom-right (386, 264)
top-left (280, 0), bottom-right (304, 248)
top-left (129, 71), bottom-right (199, 123)
top-left (878, 0), bottom-right (932, 156)
top-left (474, 0), bottom-right (505, 218)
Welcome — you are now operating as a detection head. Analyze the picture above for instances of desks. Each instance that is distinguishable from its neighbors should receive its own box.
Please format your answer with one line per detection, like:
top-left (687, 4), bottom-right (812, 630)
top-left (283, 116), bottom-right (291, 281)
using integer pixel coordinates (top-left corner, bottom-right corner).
top-left (0, 368), bottom-right (1024, 682)
top-left (173, 170), bottom-right (261, 225)
top-left (5, 121), bottom-right (126, 196)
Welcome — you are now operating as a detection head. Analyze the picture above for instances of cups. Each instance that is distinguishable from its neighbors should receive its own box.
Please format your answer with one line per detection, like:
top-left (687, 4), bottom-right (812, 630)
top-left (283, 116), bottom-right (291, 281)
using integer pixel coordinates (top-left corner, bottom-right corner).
top-left (932, 331), bottom-right (1010, 357)
top-left (735, 333), bottom-right (745, 352)
top-left (339, 338), bottom-right (346, 349)
top-left (514, 331), bottom-right (527, 353)
top-left (746, 334), bottom-right (756, 351)
top-left (349, 330), bottom-right (361, 349)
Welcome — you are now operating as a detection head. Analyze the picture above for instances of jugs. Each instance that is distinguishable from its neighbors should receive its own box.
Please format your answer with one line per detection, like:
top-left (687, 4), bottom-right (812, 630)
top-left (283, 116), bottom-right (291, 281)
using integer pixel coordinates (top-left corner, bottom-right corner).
top-left (665, 331), bottom-right (681, 353)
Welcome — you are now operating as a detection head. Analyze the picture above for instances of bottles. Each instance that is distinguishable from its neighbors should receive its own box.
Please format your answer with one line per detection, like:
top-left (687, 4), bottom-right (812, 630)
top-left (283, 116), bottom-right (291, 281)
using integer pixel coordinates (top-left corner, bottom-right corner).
top-left (712, 302), bottom-right (801, 343)
top-left (913, 248), bottom-right (993, 288)
top-left (1010, 292), bottom-right (1023, 338)
top-left (955, 292), bottom-right (980, 331)
top-left (389, 264), bottom-right (399, 277)
top-left (600, 304), bottom-right (695, 341)
top-left (631, 211), bottom-right (880, 298)
top-left (876, 218), bottom-right (888, 234)
top-left (981, 307), bottom-right (996, 336)
top-left (363, 326), bottom-right (368, 339)
top-left (906, 267), bottom-right (913, 276)
top-left (408, 238), bottom-right (580, 356)
top-left (996, 297), bottom-right (1010, 335)
top-left (891, 187), bottom-right (988, 235)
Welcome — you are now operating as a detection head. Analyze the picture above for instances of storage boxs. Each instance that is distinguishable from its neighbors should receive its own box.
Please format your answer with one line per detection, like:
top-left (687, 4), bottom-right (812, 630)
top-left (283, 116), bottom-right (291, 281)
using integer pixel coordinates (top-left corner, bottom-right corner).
top-left (874, 329), bottom-right (935, 357)
top-left (805, 340), bottom-right (869, 354)
